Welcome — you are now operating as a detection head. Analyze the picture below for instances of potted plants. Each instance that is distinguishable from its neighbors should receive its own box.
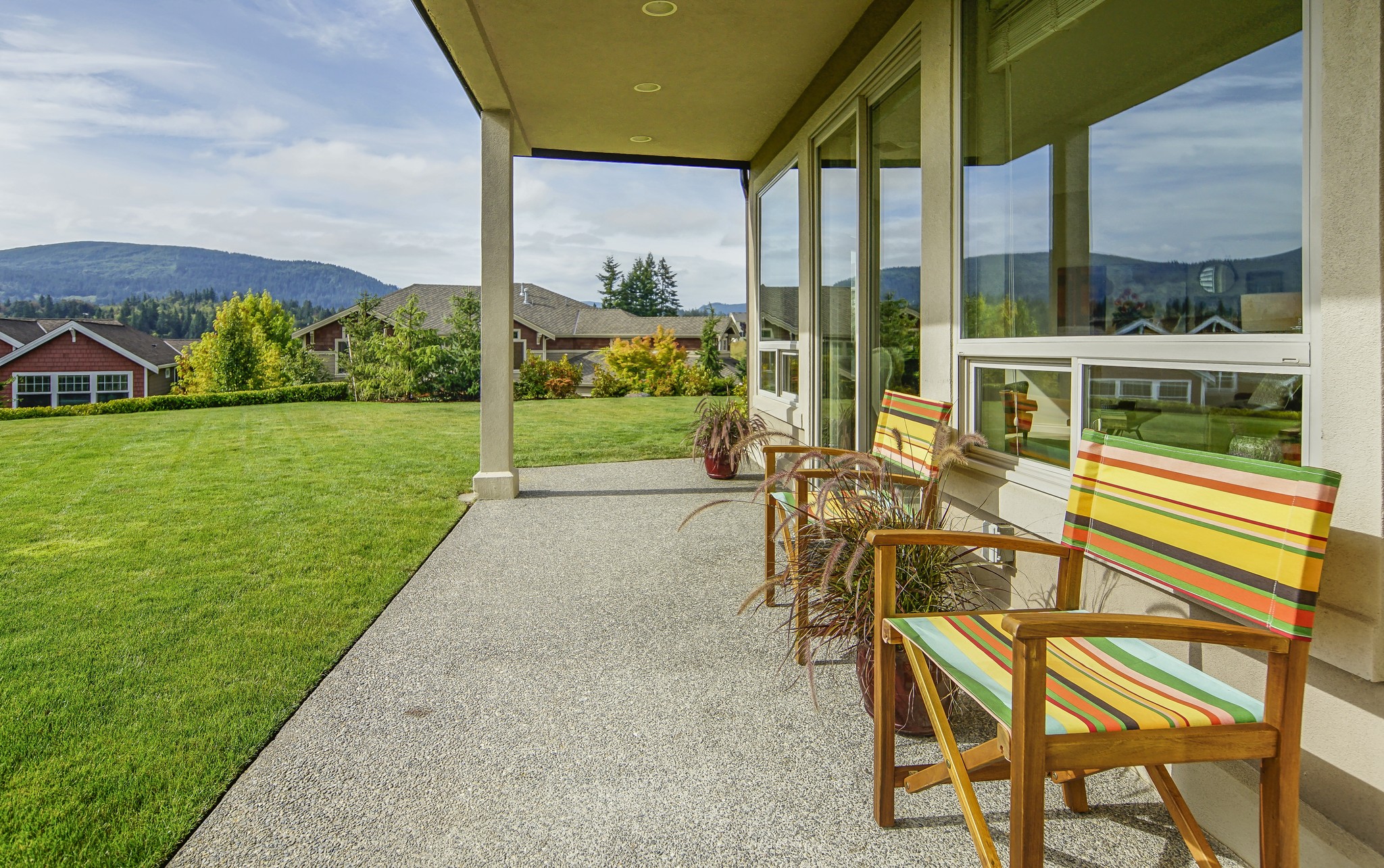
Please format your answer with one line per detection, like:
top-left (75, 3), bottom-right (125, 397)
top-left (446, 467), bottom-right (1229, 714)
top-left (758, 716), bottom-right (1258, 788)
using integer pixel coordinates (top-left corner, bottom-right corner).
top-left (680, 382), bottom-right (774, 479)
top-left (676, 427), bottom-right (1028, 734)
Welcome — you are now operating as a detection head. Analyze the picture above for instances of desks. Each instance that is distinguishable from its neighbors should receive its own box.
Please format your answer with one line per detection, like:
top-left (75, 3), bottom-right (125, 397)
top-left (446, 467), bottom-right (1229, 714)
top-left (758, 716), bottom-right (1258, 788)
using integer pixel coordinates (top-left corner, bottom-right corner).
top-left (1067, 407), bottom-right (1161, 440)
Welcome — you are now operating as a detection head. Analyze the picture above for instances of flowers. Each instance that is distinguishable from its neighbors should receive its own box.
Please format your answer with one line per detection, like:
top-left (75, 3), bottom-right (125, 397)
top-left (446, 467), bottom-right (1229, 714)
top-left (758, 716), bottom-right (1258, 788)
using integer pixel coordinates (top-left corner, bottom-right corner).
top-left (1112, 288), bottom-right (1153, 333)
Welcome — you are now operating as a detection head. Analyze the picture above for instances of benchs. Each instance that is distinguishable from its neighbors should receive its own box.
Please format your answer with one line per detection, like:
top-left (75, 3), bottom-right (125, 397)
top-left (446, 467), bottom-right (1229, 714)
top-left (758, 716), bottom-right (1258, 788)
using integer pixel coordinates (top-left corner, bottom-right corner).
top-left (761, 391), bottom-right (951, 666)
top-left (999, 389), bottom-right (1071, 468)
top-left (867, 431), bottom-right (1342, 868)
